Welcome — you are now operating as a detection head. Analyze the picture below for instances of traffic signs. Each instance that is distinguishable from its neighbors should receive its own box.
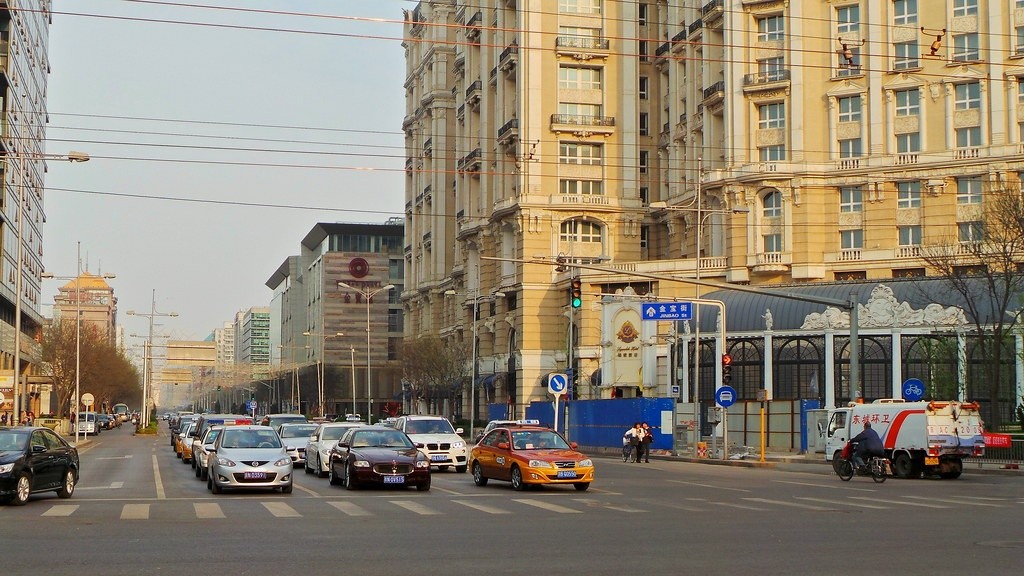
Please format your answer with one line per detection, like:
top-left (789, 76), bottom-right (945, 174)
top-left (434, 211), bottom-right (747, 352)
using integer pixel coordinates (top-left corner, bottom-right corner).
top-left (641, 302), bottom-right (692, 321)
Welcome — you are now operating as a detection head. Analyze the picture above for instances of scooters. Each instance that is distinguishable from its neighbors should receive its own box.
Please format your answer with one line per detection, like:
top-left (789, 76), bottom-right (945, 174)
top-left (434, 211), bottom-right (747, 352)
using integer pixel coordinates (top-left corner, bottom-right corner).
top-left (131, 414), bottom-right (137, 425)
top-left (838, 438), bottom-right (889, 483)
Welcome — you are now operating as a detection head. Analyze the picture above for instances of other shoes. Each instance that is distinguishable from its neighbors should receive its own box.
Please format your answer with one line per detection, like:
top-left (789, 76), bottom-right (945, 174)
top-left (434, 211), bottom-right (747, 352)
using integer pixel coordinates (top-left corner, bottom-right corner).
top-left (645, 460), bottom-right (649, 463)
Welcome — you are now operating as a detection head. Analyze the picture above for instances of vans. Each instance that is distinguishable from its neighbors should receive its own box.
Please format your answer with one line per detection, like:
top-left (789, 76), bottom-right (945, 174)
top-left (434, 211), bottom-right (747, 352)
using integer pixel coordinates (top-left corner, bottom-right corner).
top-left (346, 414), bottom-right (360, 421)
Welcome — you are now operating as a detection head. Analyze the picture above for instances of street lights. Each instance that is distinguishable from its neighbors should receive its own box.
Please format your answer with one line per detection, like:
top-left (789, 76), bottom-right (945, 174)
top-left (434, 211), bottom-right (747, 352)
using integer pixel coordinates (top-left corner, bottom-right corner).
top-left (338, 282), bottom-right (395, 425)
top-left (41, 241), bottom-right (117, 445)
top-left (126, 289), bottom-right (179, 426)
top-left (445, 290), bottom-right (506, 443)
top-left (0, 151), bottom-right (90, 428)
top-left (277, 345), bottom-right (310, 414)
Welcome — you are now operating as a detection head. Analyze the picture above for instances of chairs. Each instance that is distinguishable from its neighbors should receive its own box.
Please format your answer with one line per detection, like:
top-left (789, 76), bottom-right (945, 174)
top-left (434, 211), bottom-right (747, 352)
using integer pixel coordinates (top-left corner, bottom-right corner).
top-left (515, 439), bottom-right (531, 449)
top-left (535, 439), bottom-right (555, 448)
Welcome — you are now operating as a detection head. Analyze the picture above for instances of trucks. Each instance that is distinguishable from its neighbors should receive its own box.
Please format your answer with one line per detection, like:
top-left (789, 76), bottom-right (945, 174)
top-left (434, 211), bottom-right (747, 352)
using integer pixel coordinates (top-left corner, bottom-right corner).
top-left (818, 399), bottom-right (985, 480)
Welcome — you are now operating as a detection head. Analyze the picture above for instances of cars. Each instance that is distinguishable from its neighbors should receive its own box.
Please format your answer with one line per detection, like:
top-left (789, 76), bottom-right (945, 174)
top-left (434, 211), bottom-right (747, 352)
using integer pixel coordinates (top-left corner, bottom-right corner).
top-left (328, 427), bottom-right (432, 492)
top-left (468, 420), bottom-right (595, 491)
top-left (303, 332), bottom-right (344, 416)
top-left (163, 411), bottom-right (365, 495)
top-left (0, 426), bottom-right (80, 505)
top-left (73, 411), bottom-right (122, 435)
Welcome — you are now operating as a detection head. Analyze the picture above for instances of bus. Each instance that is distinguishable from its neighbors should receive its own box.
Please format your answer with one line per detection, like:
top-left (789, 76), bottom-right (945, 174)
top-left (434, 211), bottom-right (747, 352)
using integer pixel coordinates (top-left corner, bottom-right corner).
top-left (112, 404), bottom-right (129, 421)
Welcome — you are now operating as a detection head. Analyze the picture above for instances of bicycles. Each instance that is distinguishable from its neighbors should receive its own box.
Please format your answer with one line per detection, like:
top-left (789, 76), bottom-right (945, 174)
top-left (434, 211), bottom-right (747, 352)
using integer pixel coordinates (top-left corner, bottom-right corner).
top-left (622, 436), bottom-right (638, 463)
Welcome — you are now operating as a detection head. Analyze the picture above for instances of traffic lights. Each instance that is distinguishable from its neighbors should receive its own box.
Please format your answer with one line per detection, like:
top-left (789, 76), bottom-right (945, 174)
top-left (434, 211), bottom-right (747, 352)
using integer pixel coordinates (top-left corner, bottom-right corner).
top-left (217, 385), bottom-right (221, 391)
top-left (251, 391), bottom-right (255, 401)
top-left (722, 354), bottom-right (733, 383)
top-left (571, 277), bottom-right (582, 308)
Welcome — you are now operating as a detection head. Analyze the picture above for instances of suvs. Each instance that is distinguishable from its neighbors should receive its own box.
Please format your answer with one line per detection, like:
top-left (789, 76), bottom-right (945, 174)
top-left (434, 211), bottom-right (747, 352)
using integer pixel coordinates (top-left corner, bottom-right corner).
top-left (374, 414), bottom-right (468, 473)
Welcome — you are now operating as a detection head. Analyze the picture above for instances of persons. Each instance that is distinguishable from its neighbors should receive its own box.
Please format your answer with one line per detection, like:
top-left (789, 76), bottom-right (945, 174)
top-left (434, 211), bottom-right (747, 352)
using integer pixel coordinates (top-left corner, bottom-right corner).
top-left (62, 410), bottom-right (76, 424)
top-left (625, 421), bottom-right (652, 464)
top-left (406, 424), bottom-right (416, 433)
top-left (848, 421), bottom-right (885, 471)
top-left (15, 434), bottom-right (27, 450)
top-left (429, 424), bottom-right (440, 433)
top-left (384, 436), bottom-right (395, 446)
top-left (26, 412), bottom-right (36, 427)
top-left (536, 438), bottom-right (547, 448)
top-left (116, 415), bottom-right (123, 424)
top-left (224, 432), bottom-right (279, 447)
top-left (1, 413), bottom-right (9, 426)
